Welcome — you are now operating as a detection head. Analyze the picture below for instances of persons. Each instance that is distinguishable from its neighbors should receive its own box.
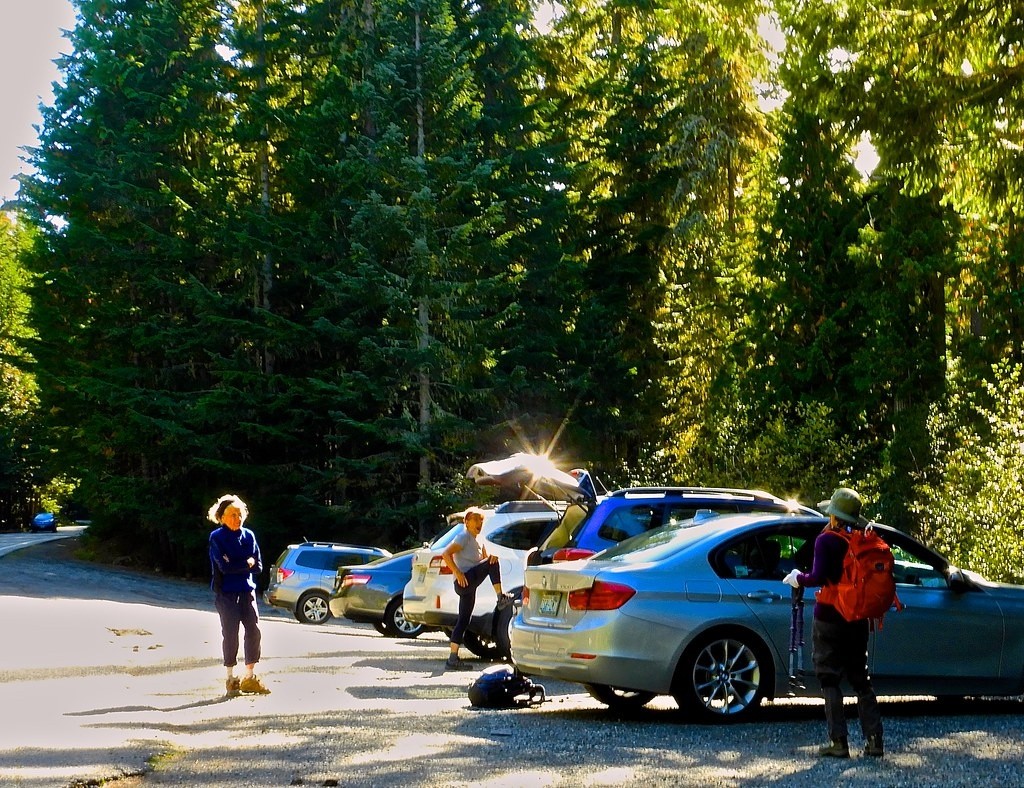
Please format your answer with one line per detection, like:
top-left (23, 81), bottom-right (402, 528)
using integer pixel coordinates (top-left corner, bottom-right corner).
top-left (783, 488), bottom-right (884, 758)
top-left (442, 507), bottom-right (523, 672)
top-left (207, 494), bottom-right (271, 694)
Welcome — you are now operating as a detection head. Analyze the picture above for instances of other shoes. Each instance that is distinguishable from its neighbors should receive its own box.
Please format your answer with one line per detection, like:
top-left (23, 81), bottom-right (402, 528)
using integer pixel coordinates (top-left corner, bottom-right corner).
top-left (226, 678), bottom-right (242, 696)
top-left (241, 676), bottom-right (272, 694)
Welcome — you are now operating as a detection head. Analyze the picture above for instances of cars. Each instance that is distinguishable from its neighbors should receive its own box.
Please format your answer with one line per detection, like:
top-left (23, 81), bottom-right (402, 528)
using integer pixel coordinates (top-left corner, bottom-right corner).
top-left (507, 510), bottom-right (1024, 727)
top-left (330, 546), bottom-right (433, 639)
top-left (32, 512), bottom-right (57, 534)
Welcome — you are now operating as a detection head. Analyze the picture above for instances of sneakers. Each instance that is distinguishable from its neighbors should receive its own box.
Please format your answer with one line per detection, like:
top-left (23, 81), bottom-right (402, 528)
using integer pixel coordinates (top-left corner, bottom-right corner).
top-left (497, 592), bottom-right (519, 610)
top-left (857, 735), bottom-right (884, 756)
top-left (446, 658), bottom-right (472, 672)
top-left (818, 736), bottom-right (848, 757)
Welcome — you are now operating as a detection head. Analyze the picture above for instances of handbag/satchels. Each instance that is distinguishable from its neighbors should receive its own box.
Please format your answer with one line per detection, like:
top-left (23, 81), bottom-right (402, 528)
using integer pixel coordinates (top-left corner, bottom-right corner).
top-left (467, 664), bottom-right (545, 707)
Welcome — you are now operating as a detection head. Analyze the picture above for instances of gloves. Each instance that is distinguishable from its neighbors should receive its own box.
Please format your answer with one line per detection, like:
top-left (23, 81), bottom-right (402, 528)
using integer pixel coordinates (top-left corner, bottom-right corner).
top-left (783, 569), bottom-right (802, 588)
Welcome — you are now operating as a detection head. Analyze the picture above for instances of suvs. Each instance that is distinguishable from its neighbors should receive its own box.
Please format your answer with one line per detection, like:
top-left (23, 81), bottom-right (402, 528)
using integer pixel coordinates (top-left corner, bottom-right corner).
top-left (402, 500), bottom-right (583, 662)
top-left (259, 541), bottom-right (393, 624)
top-left (465, 452), bottom-right (827, 573)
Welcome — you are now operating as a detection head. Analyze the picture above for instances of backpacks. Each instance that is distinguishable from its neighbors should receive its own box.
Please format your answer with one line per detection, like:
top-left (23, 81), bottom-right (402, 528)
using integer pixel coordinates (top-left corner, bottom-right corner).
top-left (815, 528), bottom-right (901, 623)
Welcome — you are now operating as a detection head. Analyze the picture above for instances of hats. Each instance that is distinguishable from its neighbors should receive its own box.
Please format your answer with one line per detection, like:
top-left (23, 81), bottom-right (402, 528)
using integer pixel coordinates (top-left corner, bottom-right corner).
top-left (818, 487), bottom-right (869, 527)
